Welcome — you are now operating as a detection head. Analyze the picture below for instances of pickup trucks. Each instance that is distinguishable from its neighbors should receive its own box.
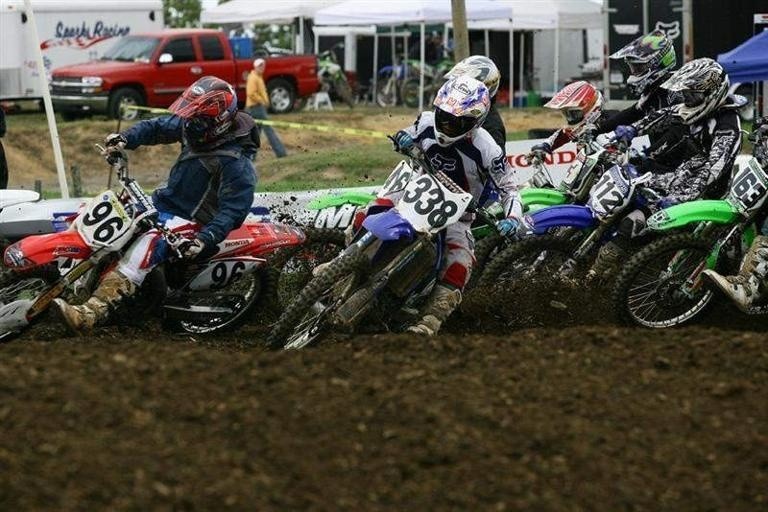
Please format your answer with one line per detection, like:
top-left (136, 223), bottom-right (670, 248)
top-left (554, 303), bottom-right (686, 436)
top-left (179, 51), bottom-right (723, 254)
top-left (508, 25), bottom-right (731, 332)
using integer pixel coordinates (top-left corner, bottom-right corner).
top-left (47, 27), bottom-right (320, 122)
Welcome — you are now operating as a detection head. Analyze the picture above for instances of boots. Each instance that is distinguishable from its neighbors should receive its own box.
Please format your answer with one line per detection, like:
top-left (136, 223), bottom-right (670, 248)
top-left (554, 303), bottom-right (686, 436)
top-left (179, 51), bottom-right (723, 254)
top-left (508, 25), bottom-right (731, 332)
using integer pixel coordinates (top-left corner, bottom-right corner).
top-left (405, 282), bottom-right (464, 338)
top-left (701, 235), bottom-right (767, 313)
top-left (51, 271), bottom-right (136, 337)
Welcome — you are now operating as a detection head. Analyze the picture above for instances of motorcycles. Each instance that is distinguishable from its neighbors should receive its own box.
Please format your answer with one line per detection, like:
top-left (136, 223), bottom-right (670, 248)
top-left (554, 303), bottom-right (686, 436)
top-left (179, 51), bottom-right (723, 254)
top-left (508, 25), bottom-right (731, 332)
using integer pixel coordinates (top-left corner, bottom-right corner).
top-left (565, 57), bottom-right (641, 100)
top-left (266, 122), bottom-right (523, 354)
top-left (462, 109), bottom-right (767, 332)
top-left (0, 141), bottom-right (306, 344)
top-left (321, 50), bottom-right (452, 110)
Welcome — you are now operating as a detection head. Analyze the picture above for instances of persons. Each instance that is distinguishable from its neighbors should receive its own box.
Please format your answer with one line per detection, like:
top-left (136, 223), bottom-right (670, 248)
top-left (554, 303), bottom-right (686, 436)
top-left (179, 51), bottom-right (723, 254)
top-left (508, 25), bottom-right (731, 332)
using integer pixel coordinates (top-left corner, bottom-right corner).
top-left (309, 74), bottom-right (528, 336)
top-left (48, 74), bottom-right (257, 338)
top-left (416, 34), bottom-right (443, 64)
top-left (243, 56), bottom-right (288, 164)
top-left (600, 56), bottom-right (750, 265)
top-left (528, 79), bottom-right (614, 164)
top-left (407, 33), bottom-right (429, 59)
top-left (442, 34), bottom-right (454, 60)
top-left (0, 104), bottom-right (10, 192)
top-left (576, 29), bottom-right (679, 146)
top-left (413, 53), bottom-right (506, 208)
top-left (698, 231), bottom-right (767, 321)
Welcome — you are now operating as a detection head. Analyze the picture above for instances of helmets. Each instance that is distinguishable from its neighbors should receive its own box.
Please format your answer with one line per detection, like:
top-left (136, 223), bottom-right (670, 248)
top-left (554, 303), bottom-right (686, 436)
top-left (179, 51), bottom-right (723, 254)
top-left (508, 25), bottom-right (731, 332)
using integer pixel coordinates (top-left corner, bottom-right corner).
top-left (168, 75), bottom-right (239, 151)
top-left (432, 54), bottom-right (502, 148)
top-left (659, 57), bottom-right (730, 127)
top-left (543, 81), bottom-right (605, 136)
top-left (609, 30), bottom-right (677, 98)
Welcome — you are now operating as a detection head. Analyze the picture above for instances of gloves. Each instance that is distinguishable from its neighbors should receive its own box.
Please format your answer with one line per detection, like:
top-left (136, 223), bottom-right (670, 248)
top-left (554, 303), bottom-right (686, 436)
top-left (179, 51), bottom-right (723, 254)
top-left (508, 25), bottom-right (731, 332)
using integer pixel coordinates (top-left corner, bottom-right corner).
top-left (177, 237), bottom-right (204, 261)
top-left (531, 143), bottom-right (551, 159)
top-left (496, 216), bottom-right (519, 238)
top-left (105, 133), bottom-right (127, 151)
top-left (615, 124), bottom-right (637, 143)
top-left (394, 130), bottom-right (415, 151)
top-left (580, 123), bottom-right (600, 140)
